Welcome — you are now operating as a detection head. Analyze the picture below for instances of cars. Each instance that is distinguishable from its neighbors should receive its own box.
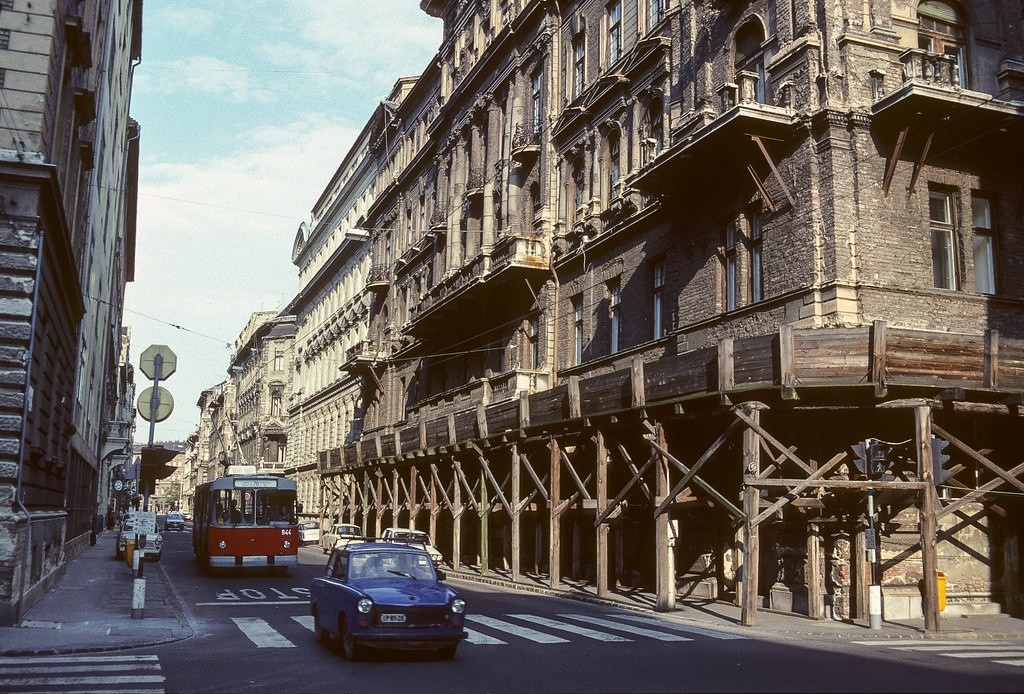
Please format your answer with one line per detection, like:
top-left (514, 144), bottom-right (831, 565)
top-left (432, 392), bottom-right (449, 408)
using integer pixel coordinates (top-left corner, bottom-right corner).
top-left (299, 522), bottom-right (320, 545)
top-left (321, 523), bottom-right (368, 553)
top-left (309, 536), bottom-right (469, 659)
top-left (119, 514), bottom-right (136, 528)
top-left (115, 519), bottom-right (163, 563)
top-left (183, 513), bottom-right (192, 519)
top-left (372, 528), bottom-right (443, 569)
top-left (165, 514), bottom-right (185, 532)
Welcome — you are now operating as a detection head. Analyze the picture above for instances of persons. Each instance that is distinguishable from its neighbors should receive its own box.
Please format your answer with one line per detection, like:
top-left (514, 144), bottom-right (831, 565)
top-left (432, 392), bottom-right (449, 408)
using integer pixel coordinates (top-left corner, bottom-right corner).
top-left (224, 499), bottom-right (242, 522)
top-left (217, 503), bottom-right (223, 518)
top-left (267, 502), bottom-right (286, 521)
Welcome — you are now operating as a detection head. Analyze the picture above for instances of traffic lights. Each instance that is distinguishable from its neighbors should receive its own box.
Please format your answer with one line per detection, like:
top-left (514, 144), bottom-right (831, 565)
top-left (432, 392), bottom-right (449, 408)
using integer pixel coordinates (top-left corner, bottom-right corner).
top-left (139, 445), bottom-right (178, 496)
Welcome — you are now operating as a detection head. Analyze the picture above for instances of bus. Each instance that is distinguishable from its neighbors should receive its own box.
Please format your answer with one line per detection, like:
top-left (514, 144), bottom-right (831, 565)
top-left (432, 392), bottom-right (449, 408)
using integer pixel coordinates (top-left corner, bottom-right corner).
top-left (192, 465), bottom-right (304, 576)
